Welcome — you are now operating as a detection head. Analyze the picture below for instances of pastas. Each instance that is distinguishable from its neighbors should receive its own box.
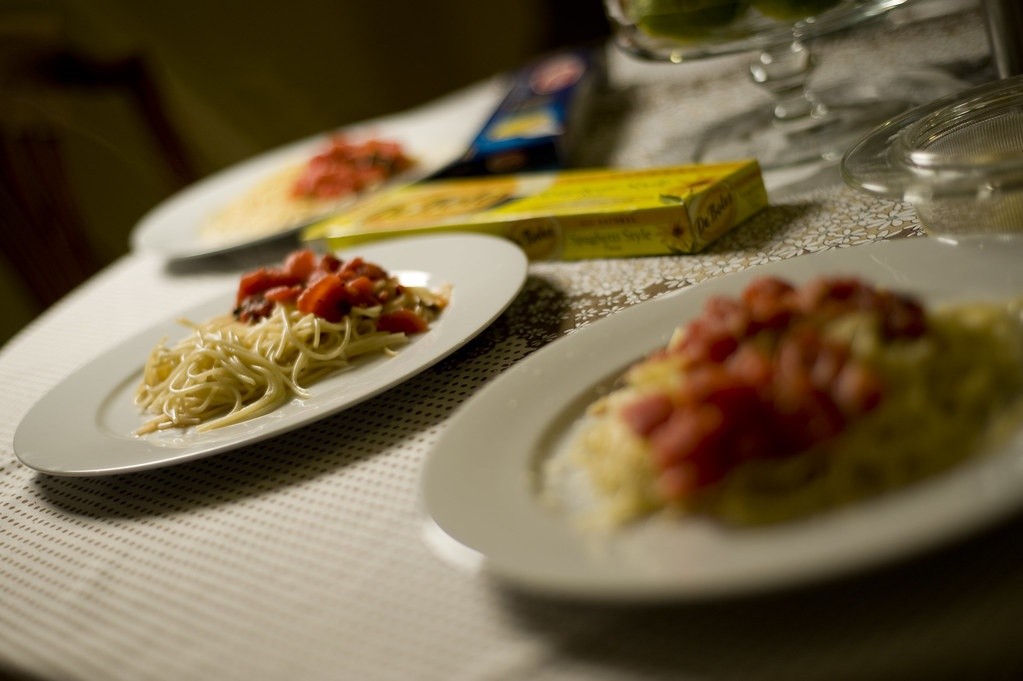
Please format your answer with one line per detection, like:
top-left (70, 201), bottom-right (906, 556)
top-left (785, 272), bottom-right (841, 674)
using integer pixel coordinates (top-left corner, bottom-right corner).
top-left (583, 316), bottom-right (976, 516)
top-left (134, 283), bottom-right (441, 429)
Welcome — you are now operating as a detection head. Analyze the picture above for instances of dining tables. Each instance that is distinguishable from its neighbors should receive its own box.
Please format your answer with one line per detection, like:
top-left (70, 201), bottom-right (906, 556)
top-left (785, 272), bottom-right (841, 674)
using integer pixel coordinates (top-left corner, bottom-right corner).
top-left (0, 19), bottom-right (1022, 679)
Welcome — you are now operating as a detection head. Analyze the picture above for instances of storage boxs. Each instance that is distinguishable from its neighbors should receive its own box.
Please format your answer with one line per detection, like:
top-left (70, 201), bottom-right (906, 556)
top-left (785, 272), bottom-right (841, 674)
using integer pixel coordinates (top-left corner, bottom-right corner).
top-left (473, 43), bottom-right (605, 170)
top-left (304, 159), bottom-right (775, 257)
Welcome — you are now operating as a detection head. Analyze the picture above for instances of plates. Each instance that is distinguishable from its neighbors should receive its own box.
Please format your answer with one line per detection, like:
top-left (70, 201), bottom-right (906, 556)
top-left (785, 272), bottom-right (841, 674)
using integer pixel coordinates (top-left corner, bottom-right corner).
top-left (415, 232), bottom-right (1022, 607)
top-left (126, 76), bottom-right (509, 264)
top-left (13, 232), bottom-right (530, 477)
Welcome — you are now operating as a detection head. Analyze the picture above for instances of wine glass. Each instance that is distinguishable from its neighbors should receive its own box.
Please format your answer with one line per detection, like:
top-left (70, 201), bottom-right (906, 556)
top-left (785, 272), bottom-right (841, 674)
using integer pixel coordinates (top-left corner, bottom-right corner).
top-left (601, 0), bottom-right (919, 177)
